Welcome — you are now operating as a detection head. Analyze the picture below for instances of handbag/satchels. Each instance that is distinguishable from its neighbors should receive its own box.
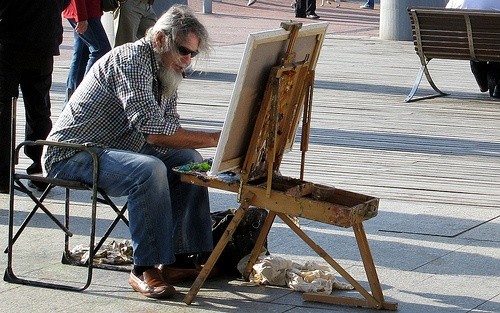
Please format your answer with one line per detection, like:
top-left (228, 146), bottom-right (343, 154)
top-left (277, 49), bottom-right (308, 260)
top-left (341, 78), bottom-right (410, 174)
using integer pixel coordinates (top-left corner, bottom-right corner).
top-left (210, 207), bottom-right (270, 280)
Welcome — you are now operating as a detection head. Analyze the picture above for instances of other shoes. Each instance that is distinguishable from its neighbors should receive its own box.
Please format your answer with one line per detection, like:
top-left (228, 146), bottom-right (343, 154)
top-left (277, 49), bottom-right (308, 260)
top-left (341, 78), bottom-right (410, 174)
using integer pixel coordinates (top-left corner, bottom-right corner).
top-left (128, 267), bottom-right (175, 297)
top-left (492, 85), bottom-right (500, 98)
top-left (306, 14), bottom-right (320, 19)
top-left (360, 5), bottom-right (374, 9)
top-left (158, 263), bottom-right (215, 282)
top-left (28, 179), bottom-right (48, 192)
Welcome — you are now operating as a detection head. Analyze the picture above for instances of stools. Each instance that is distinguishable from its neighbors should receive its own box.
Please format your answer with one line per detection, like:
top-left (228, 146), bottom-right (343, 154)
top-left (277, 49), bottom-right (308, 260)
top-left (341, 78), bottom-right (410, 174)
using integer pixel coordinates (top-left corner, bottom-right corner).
top-left (4, 96), bottom-right (133, 292)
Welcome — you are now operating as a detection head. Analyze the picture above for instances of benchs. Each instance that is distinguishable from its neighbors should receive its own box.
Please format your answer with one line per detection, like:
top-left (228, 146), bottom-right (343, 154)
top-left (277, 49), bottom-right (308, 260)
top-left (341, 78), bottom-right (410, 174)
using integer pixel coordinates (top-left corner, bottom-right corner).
top-left (403, 6), bottom-right (500, 103)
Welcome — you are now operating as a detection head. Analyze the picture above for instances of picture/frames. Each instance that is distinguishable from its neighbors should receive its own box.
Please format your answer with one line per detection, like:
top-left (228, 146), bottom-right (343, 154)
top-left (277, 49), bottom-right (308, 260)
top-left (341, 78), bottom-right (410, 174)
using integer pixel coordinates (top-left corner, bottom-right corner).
top-left (209, 21), bottom-right (329, 176)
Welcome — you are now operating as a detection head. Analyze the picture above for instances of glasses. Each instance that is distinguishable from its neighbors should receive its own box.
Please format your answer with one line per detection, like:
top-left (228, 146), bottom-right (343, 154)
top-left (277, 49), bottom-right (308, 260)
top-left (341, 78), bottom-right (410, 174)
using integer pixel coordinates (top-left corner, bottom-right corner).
top-left (175, 42), bottom-right (199, 58)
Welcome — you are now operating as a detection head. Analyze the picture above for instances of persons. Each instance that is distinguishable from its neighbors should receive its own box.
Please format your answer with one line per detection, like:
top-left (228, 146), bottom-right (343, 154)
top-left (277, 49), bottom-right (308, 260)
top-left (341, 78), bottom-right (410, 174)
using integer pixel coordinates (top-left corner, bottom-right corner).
top-left (41, 4), bottom-right (223, 300)
top-left (247, 0), bottom-right (374, 20)
top-left (152, 0), bottom-right (190, 78)
top-left (61, 0), bottom-right (120, 111)
top-left (445, 0), bottom-right (500, 97)
top-left (0, 0), bottom-right (70, 195)
top-left (111, 0), bottom-right (158, 47)
